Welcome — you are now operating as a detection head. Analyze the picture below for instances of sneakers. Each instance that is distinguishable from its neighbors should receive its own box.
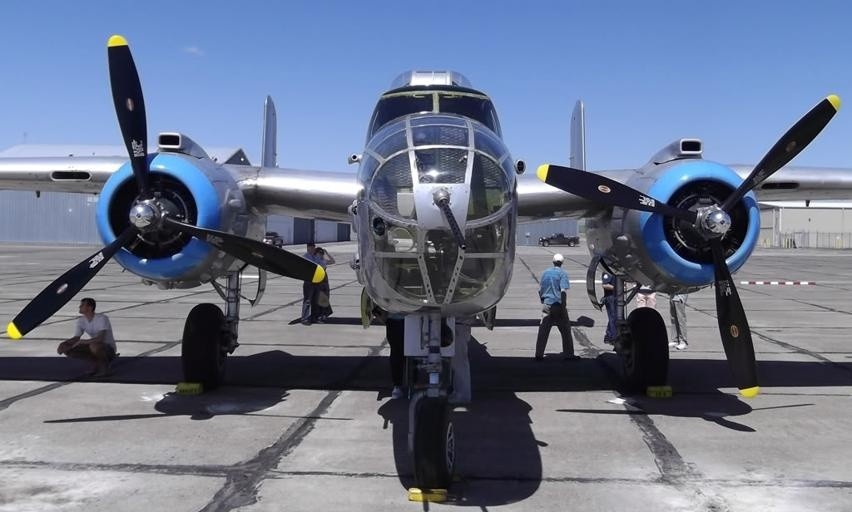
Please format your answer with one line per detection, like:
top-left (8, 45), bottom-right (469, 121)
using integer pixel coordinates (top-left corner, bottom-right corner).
top-left (669, 341), bottom-right (687, 350)
top-left (83, 367), bottom-right (116, 376)
top-left (535, 357), bottom-right (545, 360)
top-left (564, 356), bottom-right (581, 360)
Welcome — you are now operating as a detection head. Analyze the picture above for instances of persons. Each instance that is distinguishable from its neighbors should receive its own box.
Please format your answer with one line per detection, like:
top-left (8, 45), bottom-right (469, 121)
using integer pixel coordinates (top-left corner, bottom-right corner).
top-left (535, 254), bottom-right (581, 360)
top-left (57, 298), bottom-right (117, 378)
top-left (601, 270), bottom-right (617, 345)
top-left (669, 293), bottom-right (688, 350)
top-left (633, 283), bottom-right (657, 310)
top-left (301, 242), bottom-right (335, 326)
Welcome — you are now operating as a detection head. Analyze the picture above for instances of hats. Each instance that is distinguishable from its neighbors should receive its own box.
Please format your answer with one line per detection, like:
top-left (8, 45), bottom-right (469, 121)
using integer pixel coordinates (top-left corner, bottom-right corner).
top-left (553, 254), bottom-right (563, 261)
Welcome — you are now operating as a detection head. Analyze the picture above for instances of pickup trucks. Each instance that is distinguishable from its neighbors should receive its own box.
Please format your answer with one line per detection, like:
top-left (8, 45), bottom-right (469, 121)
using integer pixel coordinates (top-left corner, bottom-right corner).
top-left (263, 232), bottom-right (284, 248)
top-left (539, 232), bottom-right (580, 248)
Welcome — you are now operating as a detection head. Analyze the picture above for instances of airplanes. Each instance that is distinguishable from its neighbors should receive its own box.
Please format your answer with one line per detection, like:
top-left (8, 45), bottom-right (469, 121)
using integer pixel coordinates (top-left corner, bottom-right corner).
top-left (0, 33), bottom-right (852, 490)
